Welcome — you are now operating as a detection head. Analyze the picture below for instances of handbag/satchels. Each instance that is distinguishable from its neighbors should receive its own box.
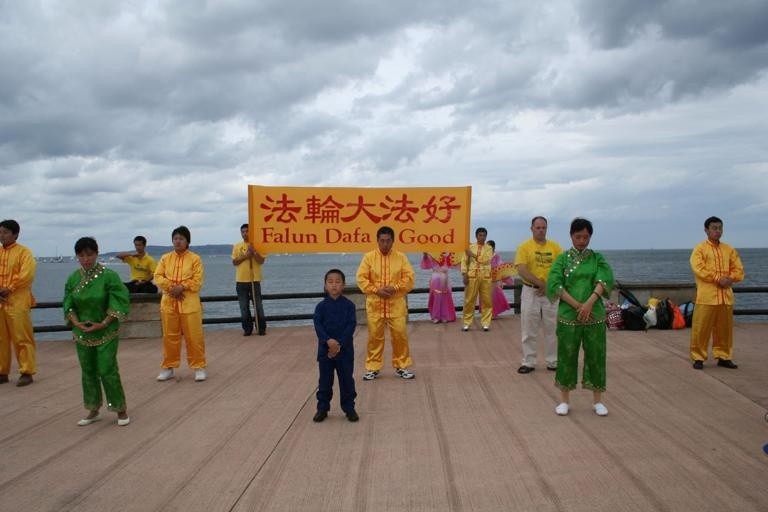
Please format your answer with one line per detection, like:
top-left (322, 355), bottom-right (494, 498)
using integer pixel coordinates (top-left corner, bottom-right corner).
top-left (678, 300), bottom-right (694, 327)
top-left (669, 301), bottom-right (686, 329)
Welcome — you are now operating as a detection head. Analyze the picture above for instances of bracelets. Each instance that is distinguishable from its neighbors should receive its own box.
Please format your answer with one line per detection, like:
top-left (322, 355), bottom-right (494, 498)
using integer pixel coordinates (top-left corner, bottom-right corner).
top-left (102, 321), bottom-right (108, 329)
top-left (591, 289), bottom-right (601, 300)
top-left (252, 251), bottom-right (257, 255)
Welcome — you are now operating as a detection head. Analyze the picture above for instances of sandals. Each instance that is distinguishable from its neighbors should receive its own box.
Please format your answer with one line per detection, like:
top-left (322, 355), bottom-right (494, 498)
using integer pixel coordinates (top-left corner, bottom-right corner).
top-left (518, 366), bottom-right (535, 373)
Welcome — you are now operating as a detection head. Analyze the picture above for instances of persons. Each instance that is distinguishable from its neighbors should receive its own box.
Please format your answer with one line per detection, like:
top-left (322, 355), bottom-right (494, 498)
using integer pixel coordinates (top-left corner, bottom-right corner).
top-left (689, 214), bottom-right (747, 369)
top-left (356, 226), bottom-right (416, 380)
top-left (62, 236), bottom-right (131, 427)
top-left (420, 226), bottom-right (514, 329)
top-left (231, 224), bottom-right (266, 336)
top-left (153, 225), bottom-right (208, 381)
top-left (1, 219), bottom-right (38, 386)
top-left (546, 217), bottom-right (616, 416)
top-left (116, 235), bottom-right (158, 294)
top-left (513, 216), bottom-right (563, 374)
top-left (312, 269), bottom-right (359, 422)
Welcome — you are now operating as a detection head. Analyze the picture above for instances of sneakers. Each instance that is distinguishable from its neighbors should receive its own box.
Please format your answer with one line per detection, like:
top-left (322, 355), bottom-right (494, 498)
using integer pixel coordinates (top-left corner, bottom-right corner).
top-left (156, 368), bottom-right (174, 381)
top-left (433, 318), bottom-right (489, 331)
top-left (313, 410), bottom-right (328, 421)
top-left (16, 374), bottom-right (33, 386)
top-left (195, 368), bottom-right (207, 380)
top-left (394, 368), bottom-right (416, 378)
top-left (362, 370), bottom-right (383, 380)
top-left (345, 410), bottom-right (360, 422)
top-left (591, 401), bottom-right (607, 416)
top-left (692, 356), bottom-right (738, 369)
top-left (555, 402), bottom-right (570, 415)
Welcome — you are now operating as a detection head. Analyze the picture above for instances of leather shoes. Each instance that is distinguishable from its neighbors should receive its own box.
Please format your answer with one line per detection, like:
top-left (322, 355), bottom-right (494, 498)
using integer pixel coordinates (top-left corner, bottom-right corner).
top-left (117, 412), bottom-right (129, 425)
top-left (77, 415), bottom-right (102, 424)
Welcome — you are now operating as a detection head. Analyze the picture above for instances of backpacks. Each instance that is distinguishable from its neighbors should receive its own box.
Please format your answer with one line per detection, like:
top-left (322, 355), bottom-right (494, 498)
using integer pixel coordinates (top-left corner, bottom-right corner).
top-left (622, 307), bottom-right (646, 330)
top-left (656, 299), bottom-right (673, 330)
